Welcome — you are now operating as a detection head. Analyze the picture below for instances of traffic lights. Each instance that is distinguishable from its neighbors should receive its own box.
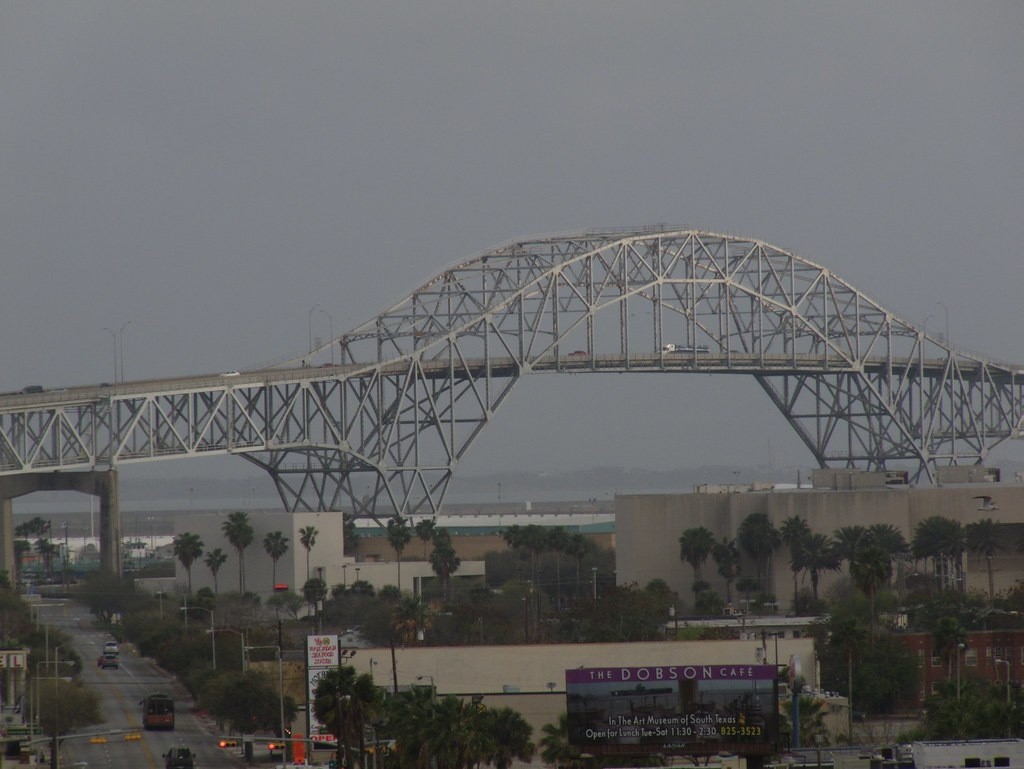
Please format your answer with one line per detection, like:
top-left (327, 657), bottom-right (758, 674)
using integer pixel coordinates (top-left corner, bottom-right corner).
top-left (269, 742), bottom-right (286, 751)
top-left (364, 748), bottom-right (374, 754)
top-left (123, 733), bottom-right (141, 742)
top-left (220, 741), bottom-right (238, 748)
top-left (91, 737), bottom-right (107, 745)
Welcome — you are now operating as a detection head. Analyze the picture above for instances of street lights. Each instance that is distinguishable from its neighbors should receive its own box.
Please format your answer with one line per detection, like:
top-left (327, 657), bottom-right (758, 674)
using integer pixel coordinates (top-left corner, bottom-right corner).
top-left (53, 640), bottom-right (97, 769)
top-left (30, 676), bottom-right (72, 756)
top-left (244, 646), bottom-right (286, 769)
top-left (957, 644), bottom-right (965, 697)
top-left (318, 309), bottom-right (335, 368)
top-left (996, 658), bottom-right (1011, 708)
top-left (35, 660), bottom-right (76, 726)
top-left (180, 605), bottom-right (216, 670)
top-left (206, 628), bottom-right (245, 680)
top-left (120, 320), bottom-right (133, 384)
top-left (309, 303), bottom-right (321, 367)
top-left (46, 618), bottom-right (81, 673)
top-left (102, 326), bottom-right (117, 385)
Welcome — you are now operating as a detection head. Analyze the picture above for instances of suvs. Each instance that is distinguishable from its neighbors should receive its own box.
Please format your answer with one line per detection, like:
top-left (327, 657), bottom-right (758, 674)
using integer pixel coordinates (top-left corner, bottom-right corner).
top-left (16, 386), bottom-right (42, 395)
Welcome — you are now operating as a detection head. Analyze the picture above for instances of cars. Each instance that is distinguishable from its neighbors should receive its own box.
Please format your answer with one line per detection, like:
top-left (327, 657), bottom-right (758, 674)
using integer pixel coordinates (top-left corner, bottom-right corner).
top-left (568, 350), bottom-right (587, 356)
top-left (218, 371), bottom-right (241, 379)
top-left (162, 747), bottom-right (197, 769)
top-left (101, 654), bottom-right (119, 670)
top-left (103, 640), bottom-right (118, 655)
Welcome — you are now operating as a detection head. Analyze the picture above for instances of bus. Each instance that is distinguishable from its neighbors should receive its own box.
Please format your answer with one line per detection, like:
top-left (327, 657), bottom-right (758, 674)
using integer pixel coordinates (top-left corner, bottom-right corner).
top-left (138, 691), bottom-right (174, 729)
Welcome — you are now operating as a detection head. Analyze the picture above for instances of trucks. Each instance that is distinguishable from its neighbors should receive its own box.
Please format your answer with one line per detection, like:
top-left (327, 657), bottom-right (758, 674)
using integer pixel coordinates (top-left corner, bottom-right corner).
top-left (658, 343), bottom-right (712, 354)
